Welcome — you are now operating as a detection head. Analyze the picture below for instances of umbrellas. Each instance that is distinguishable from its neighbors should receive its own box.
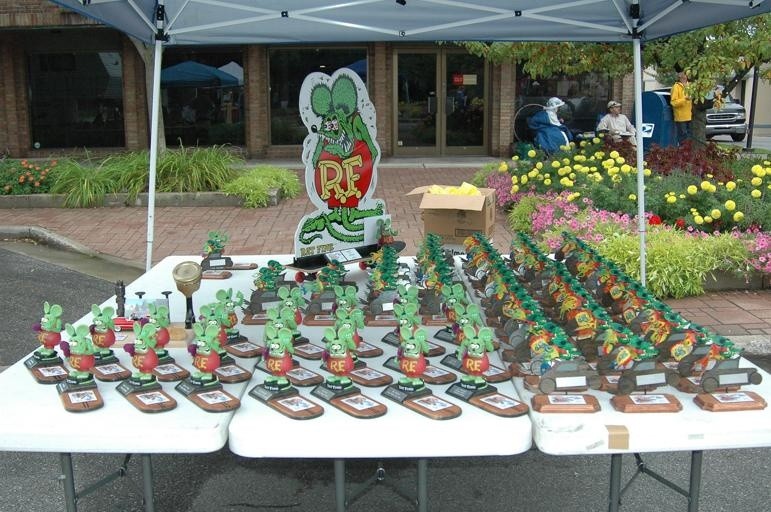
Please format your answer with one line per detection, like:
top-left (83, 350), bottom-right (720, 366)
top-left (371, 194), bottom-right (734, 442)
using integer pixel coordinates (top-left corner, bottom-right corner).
top-left (161, 60), bottom-right (238, 98)
top-left (217, 61), bottom-right (243, 89)
top-left (346, 60), bottom-right (368, 73)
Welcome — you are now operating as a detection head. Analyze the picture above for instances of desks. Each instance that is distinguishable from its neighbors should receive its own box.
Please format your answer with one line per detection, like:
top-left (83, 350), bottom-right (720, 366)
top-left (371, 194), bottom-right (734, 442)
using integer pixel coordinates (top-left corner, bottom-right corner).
top-left (228, 255), bottom-right (534, 512)
top-left (452, 253), bottom-right (771, 512)
top-left (0, 255), bottom-right (296, 512)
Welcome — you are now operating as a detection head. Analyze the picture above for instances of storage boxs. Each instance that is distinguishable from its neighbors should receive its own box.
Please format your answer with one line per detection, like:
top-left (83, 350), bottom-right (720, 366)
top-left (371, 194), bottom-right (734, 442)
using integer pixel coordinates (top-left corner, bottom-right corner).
top-left (405, 184), bottom-right (496, 254)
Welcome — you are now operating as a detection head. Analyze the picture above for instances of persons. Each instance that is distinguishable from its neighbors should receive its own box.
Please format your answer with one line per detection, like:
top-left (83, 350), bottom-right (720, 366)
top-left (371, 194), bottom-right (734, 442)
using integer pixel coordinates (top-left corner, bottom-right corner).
top-left (190, 88), bottom-right (216, 123)
top-left (280, 82), bottom-right (289, 110)
top-left (209, 77), bottom-right (221, 104)
top-left (456, 85), bottom-right (467, 103)
top-left (93, 104), bottom-right (121, 128)
top-left (223, 91), bottom-right (232, 102)
top-left (671, 72), bottom-right (693, 144)
top-left (163, 100), bottom-right (193, 146)
top-left (527, 96), bottom-right (573, 153)
top-left (596, 102), bottom-right (637, 151)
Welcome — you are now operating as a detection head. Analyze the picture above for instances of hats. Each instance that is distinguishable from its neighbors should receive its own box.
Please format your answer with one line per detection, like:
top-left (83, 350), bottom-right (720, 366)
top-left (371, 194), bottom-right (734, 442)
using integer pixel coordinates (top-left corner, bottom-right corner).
top-left (607, 101), bottom-right (622, 109)
top-left (543, 97), bottom-right (566, 110)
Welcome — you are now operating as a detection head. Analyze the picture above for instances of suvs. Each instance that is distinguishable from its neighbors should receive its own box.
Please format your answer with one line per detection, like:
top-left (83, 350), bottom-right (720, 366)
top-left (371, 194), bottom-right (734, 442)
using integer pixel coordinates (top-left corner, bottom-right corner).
top-left (650, 81), bottom-right (748, 145)
